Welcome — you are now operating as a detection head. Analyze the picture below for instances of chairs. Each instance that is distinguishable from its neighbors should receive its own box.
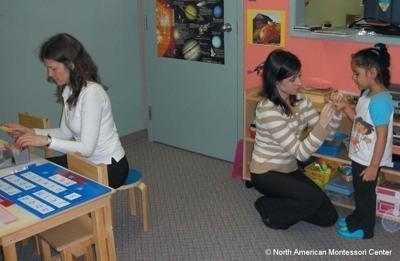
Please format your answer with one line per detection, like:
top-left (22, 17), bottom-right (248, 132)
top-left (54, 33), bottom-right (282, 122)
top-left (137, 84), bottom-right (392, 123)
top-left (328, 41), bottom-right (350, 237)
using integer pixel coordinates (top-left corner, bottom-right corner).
top-left (0, 110), bottom-right (118, 261)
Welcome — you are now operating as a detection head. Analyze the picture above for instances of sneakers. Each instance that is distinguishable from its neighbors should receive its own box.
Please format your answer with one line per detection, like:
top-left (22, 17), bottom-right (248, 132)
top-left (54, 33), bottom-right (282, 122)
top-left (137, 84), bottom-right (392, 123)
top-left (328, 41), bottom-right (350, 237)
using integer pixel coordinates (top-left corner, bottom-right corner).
top-left (337, 217), bottom-right (348, 225)
top-left (336, 226), bottom-right (374, 239)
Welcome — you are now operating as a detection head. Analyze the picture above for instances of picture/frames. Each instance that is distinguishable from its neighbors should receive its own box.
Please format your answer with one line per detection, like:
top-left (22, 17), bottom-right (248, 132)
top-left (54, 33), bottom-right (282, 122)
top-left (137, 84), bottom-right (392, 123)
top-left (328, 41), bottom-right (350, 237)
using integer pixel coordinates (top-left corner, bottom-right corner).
top-left (245, 8), bottom-right (286, 49)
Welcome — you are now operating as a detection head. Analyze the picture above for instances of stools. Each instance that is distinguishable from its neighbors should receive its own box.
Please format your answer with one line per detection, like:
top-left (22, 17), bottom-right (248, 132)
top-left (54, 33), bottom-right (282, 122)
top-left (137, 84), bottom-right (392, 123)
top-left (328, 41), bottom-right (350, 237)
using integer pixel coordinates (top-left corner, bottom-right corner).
top-left (117, 168), bottom-right (149, 232)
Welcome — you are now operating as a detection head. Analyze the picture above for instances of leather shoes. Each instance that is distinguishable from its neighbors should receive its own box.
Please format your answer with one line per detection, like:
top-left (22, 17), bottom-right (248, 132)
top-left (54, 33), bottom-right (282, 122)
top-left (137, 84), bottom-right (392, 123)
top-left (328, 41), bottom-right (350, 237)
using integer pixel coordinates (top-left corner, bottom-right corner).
top-left (253, 196), bottom-right (282, 229)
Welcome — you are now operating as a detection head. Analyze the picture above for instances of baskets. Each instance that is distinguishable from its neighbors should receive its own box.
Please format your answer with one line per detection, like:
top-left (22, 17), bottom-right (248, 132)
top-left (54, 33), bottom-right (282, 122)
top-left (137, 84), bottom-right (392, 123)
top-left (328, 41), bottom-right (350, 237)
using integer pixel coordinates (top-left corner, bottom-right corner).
top-left (303, 157), bottom-right (345, 184)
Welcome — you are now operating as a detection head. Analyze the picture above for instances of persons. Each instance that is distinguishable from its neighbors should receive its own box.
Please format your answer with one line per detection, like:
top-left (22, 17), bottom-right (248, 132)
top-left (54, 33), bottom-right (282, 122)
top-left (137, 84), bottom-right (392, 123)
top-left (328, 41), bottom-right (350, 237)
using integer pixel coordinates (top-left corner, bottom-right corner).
top-left (3, 33), bottom-right (129, 244)
top-left (327, 43), bottom-right (395, 241)
top-left (249, 51), bottom-right (338, 231)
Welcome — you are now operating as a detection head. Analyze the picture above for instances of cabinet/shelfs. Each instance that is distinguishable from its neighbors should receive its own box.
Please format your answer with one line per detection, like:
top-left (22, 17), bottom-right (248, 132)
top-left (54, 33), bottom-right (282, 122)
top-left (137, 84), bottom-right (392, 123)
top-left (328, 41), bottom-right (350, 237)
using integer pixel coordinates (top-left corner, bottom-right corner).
top-left (242, 86), bottom-right (400, 223)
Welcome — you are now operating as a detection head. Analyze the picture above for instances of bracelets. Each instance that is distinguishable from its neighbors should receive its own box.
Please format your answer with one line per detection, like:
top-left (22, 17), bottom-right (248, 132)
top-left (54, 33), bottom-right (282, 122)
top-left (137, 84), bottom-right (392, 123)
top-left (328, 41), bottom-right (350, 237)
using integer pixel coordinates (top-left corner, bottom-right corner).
top-left (46, 135), bottom-right (52, 147)
